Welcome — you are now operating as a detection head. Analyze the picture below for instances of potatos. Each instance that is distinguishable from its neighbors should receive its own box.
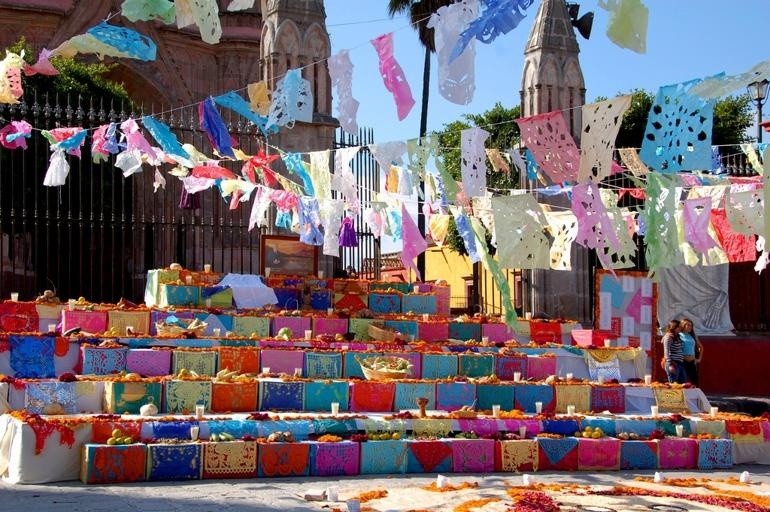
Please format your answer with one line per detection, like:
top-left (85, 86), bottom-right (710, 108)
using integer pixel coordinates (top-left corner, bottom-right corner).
top-left (211, 431), bottom-right (235, 442)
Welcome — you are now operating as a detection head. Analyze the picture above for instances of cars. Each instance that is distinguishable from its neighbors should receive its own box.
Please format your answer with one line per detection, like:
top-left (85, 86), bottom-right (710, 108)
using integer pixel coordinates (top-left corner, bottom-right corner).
top-left (318, 270), bottom-right (324, 279)
top-left (675, 425), bottom-right (683, 438)
top-left (535, 401), bottom-right (542, 414)
top-left (526, 312), bottom-right (531, 320)
top-left (186, 275), bottom-right (193, 285)
top-left (513, 372), bottom-right (521, 383)
top-left (482, 336), bottom-right (488, 347)
top-left (213, 327), bottom-right (222, 337)
top-left (195, 404), bottom-right (204, 419)
top-left (651, 405), bottom-right (659, 416)
top-left (328, 484), bottom-right (338, 501)
top-left (11, 293), bottom-right (75, 333)
top-left (519, 425), bottom-right (526, 439)
top-left (437, 474), bottom-right (446, 488)
top-left (191, 425), bottom-right (200, 442)
top-left (328, 308), bottom-right (333, 317)
top-left (567, 404), bottom-right (576, 414)
top-left (305, 329), bottom-right (311, 341)
top-left (204, 264), bottom-right (211, 273)
top-left (265, 267), bottom-right (271, 278)
top-left (423, 313), bottom-right (429, 321)
top-left (711, 407), bottom-right (719, 418)
top-left (644, 374), bottom-right (651, 384)
top-left (331, 402), bottom-right (338, 416)
top-left (493, 405), bottom-right (500, 417)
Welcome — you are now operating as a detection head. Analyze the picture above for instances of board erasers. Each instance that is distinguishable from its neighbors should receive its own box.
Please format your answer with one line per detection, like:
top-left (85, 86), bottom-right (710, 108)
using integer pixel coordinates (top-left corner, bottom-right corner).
top-left (618, 432), bottom-right (629, 440)
top-left (630, 433), bottom-right (638, 439)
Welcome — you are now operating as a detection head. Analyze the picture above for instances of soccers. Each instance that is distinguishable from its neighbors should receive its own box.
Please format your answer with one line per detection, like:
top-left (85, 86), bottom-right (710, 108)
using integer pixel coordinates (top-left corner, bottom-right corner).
top-left (369, 432), bottom-right (401, 440)
top-left (573, 426), bottom-right (604, 438)
top-left (107, 429), bottom-right (133, 445)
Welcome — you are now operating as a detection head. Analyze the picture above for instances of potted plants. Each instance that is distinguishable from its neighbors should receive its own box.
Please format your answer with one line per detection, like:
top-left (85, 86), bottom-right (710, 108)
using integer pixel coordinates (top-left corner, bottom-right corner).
top-left (259, 232), bottom-right (319, 278)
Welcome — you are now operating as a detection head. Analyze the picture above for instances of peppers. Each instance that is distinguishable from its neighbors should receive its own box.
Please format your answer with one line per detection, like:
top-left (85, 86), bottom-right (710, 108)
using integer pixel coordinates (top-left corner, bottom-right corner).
top-left (35, 290), bottom-right (61, 303)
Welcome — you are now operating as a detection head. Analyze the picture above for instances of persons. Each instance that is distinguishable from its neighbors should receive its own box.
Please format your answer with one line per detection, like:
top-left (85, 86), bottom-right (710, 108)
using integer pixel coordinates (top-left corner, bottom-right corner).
top-left (664, 319), bottom-right (688, 384)
top-left (678, 319), bottom-right (704, 388)
